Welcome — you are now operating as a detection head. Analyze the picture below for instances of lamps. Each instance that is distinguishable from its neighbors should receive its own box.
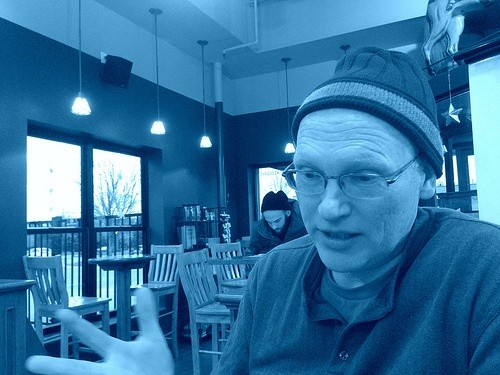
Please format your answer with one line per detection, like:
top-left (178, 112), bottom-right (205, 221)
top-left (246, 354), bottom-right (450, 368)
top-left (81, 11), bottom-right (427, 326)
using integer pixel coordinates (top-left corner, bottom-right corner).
top-left (281, 57), bottom-right (296, 154)
top-left (70, 0), bottom-right (91, 117)
top-left (196, 39), bottom-right (213, 148)
top-left (147, 7), bottom-right (167, 135)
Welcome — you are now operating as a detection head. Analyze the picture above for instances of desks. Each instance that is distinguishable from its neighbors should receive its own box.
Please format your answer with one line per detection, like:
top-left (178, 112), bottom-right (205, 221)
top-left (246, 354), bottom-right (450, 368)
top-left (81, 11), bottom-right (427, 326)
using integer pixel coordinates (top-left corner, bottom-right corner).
top-left (85, 253), bottom-right (158, 341)
top-left (208, 254), bottom-right (266, 267)
top-left (214, 278), bottom-right (251, 333)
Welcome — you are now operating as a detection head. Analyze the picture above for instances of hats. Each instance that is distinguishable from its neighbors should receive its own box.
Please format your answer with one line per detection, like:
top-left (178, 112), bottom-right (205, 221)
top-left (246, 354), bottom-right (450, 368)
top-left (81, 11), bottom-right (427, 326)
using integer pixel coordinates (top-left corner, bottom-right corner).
top-left (260, 191), bottom-right (290, 212)
top-left (290, 46), bottom-right (443, 179)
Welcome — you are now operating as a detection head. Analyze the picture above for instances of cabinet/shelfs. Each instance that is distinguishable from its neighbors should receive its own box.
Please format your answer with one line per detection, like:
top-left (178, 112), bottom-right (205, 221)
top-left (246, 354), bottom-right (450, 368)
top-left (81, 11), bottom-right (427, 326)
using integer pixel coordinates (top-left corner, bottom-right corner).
top-left (173, 207), bottom-right (231, 253)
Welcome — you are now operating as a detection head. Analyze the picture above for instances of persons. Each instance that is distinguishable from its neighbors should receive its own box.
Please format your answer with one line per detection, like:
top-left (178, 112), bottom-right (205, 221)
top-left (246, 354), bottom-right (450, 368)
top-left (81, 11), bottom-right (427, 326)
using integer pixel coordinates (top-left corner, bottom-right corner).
top-left (250, 191), bottom-right (307, 255)
top-left (25, 47), bottom-right (500, 375)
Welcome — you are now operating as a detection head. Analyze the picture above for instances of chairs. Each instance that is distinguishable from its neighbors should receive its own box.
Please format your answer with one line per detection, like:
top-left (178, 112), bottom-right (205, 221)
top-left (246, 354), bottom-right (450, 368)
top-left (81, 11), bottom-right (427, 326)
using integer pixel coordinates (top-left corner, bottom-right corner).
top-left (22, 253), bottom-right (113, 360)
top-left (130, 244), bottom-right (185, 358)
top-left (174, 248), bottom-right (230, 375)
top-left (209, 240), bottom-right (244, 295)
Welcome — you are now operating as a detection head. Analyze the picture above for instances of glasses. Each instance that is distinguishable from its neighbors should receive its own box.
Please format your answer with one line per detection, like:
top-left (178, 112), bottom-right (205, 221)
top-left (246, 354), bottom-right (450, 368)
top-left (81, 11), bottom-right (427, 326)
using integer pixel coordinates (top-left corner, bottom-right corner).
top-left (282, 150), bottom-right (421, 200)
